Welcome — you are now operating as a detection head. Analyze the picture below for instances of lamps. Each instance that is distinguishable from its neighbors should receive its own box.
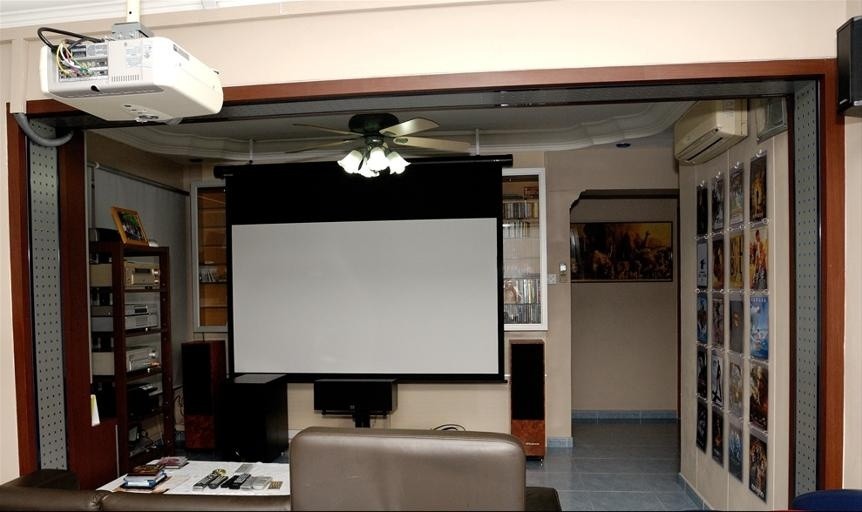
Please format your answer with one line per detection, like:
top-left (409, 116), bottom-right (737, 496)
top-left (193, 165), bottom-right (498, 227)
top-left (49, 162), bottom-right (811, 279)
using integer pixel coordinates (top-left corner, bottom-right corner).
top-left (336, 146), bottom-right (411, 179)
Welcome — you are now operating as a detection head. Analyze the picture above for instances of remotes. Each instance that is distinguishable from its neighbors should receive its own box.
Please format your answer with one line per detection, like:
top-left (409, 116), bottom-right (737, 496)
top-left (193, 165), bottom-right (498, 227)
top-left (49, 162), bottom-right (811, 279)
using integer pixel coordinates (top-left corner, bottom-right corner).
top-left (192, 464), bottom-right (272, 490)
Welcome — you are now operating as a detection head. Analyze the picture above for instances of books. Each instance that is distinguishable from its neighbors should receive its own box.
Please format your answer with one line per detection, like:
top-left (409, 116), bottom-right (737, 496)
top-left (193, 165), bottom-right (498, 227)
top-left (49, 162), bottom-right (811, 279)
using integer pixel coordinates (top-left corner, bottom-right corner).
top-left (126, 470), bottom-right (163, 479)
top-left (158, 455), bottom-right (189, 470)
top-left (128, 464), bottom-right (166, 474)
top-left (503, 185), bottom-right (539, 237)
top-left (128, 472), bottom-right (167, 486)
top-left (503, 272), bottom-right (542, 325)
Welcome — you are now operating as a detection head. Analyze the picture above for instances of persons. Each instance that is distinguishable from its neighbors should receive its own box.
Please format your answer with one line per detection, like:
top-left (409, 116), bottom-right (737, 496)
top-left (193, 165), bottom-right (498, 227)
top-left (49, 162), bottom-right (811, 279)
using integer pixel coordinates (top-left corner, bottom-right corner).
top-left (748, 363), bottom-right (768, 411)
top-left (754, 186), bottom-right (760, 206)
top-left (752, 228), bottom-right (762, 265)
top-left (715, 359), bottom-right (721, 397)
top-left (697, 351), bottom-right (707, 380)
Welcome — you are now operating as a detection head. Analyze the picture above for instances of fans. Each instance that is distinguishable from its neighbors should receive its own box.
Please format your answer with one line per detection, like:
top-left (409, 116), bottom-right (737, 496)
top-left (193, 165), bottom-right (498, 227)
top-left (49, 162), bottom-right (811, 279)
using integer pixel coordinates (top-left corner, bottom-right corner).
top-left (285, 114), bottom-right (472, 179)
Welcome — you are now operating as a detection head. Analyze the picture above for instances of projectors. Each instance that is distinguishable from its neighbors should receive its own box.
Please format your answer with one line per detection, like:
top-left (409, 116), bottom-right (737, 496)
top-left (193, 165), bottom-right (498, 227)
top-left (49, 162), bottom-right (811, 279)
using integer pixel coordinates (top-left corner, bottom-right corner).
top-left (39, 21), bottom-right (224, 123)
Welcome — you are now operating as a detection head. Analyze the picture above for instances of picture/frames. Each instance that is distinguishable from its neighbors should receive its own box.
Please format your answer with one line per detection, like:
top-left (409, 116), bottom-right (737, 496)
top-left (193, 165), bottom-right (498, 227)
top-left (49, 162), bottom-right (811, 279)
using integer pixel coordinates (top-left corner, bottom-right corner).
top-left (569, 220), bottom-right (674, 284)
top-left (110, 206), bottom-right (150, 247)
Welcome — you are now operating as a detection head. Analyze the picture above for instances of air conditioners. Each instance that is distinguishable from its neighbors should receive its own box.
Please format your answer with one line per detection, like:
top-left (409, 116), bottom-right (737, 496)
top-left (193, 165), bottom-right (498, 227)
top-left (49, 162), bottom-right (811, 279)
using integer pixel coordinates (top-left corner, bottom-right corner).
top-left (673, 99), bottom-right (748, 167)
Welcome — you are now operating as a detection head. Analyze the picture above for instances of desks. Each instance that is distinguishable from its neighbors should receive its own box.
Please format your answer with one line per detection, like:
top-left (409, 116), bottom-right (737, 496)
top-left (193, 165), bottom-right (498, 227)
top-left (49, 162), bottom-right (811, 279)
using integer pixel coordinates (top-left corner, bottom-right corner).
top-left (95, 457), bottom-right (290, 496)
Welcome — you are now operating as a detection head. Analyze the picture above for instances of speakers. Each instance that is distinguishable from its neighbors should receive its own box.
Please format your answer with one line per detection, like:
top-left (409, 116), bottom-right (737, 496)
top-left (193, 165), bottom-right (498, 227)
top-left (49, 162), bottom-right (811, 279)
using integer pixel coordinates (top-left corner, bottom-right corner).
top-left (181, 339), bottom-right (227, 457)
top-left (509, 339), bottom-right (546, 460)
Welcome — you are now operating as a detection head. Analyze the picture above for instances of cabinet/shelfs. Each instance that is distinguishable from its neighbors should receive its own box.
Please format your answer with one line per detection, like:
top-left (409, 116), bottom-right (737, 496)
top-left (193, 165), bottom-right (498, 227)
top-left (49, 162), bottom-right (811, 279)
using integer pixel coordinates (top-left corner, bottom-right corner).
top-left (89, 244), bottom-right (176, 479)
top-left (501, 167), bottom-right (548, 332)
top-left (190, 179), bottom-right (229, 334)
